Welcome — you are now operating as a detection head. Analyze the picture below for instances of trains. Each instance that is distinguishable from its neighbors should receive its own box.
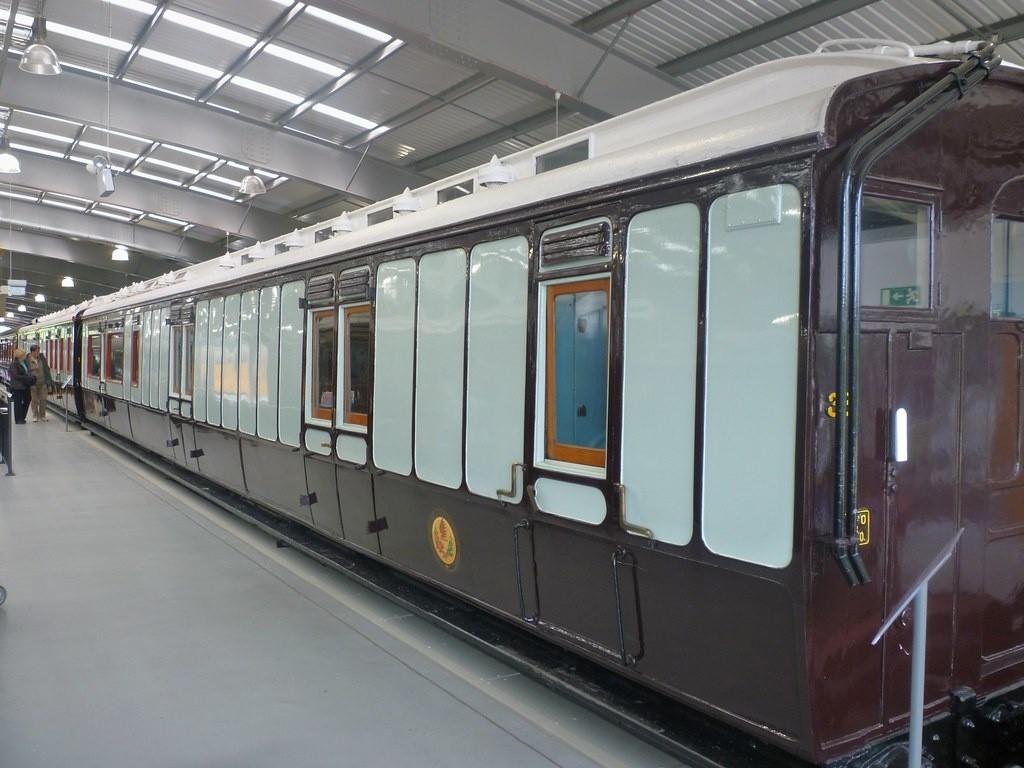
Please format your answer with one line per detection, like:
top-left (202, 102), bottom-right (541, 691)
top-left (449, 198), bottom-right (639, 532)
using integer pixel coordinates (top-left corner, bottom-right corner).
top-left (16, 35), bottom-right (1024, 767)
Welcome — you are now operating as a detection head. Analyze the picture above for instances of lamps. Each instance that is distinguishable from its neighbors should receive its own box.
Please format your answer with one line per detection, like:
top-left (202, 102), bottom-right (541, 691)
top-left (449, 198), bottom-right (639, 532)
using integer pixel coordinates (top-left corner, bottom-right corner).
top-left (238, 168), bottom-right (266, 196)
top-left (18, 0), bottom-right (65, 75)
top-left (0, 145), bottom-right (21, 175)
top-left (86, 156), bottom-right (115, 195)
top-left (110, 244), bottom-right (130, 262)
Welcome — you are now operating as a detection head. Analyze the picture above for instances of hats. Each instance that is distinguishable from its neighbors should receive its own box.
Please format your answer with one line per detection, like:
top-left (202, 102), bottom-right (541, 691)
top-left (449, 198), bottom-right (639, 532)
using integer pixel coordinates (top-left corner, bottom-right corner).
top-left (14, 349), bottom-right (26, 358)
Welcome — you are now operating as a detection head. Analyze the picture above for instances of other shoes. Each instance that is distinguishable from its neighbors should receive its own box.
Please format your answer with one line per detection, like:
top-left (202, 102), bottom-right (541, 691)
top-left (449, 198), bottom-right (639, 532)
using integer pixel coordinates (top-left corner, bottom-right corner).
top-left (42, 417), bottom-right (49, 421)
top-left (32, 417), bottom-right (37, 422)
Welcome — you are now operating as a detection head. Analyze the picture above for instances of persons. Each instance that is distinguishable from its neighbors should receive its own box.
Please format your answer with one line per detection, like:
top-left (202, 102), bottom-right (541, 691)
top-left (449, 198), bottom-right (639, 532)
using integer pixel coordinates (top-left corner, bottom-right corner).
top-left (8, 348), bottom-right (37, 424)
top-left (89, 351), bottom-right (119, 378)
top-left (25, 345), bottom-right (53, 422)
